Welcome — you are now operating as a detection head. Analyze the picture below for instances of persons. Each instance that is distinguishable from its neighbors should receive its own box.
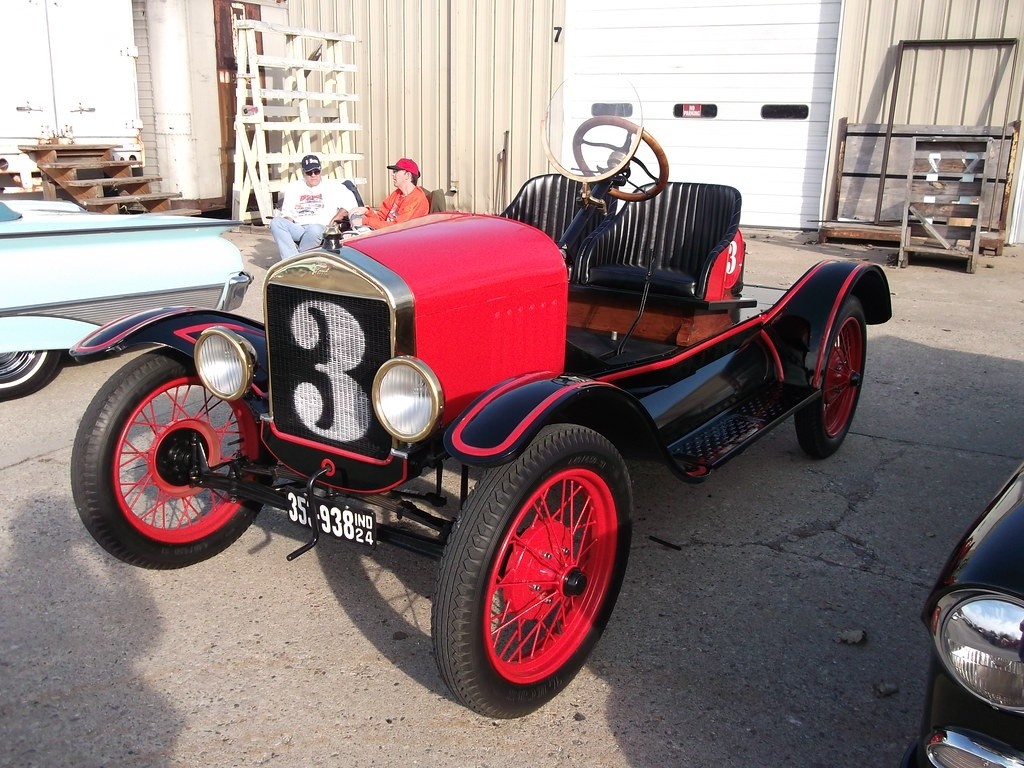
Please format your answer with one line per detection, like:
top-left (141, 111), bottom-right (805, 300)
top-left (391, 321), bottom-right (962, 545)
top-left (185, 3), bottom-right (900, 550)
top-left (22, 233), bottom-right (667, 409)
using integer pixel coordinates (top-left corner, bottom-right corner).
top-left (348, 158), bottom-right (429, 230)
top-left (270, 155), bottom-right (358, 259)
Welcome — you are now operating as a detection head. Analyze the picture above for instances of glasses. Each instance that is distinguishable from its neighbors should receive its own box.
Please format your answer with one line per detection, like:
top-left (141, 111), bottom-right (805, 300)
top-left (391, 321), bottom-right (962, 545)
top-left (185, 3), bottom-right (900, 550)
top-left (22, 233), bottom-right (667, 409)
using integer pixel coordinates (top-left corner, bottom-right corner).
top-left (392, 170), bottom-right (399, 173)
top-left (303, 169), bottom-right (321, 176)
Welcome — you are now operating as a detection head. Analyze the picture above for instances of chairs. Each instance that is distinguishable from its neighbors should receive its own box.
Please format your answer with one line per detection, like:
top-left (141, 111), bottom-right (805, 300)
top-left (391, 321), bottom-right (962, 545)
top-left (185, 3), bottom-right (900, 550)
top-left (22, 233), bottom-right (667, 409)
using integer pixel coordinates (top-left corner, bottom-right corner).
top-left (572, 174), bottom-right (743, 298)
top-left (498, 173), bottom-right (617, 269)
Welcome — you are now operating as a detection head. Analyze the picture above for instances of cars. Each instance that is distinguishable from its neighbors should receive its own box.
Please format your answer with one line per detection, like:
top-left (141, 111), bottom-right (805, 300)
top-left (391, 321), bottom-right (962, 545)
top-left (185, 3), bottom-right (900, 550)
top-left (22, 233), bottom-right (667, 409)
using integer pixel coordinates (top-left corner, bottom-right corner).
top-left (69, 65), bottom-right (893, 720)
top-left (899, 464), bottom-right (1024, 768)
top-left (0, 197), bottom-right (255, 403)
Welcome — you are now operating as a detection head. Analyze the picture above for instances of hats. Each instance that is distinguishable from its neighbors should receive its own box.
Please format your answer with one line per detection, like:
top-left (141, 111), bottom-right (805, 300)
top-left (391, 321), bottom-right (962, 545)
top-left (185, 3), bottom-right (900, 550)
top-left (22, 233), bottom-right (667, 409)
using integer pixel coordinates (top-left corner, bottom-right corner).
top-left (302, 155), bottom-right (321, 172)
top-left (387, 158), bottom-right (418, 176)
